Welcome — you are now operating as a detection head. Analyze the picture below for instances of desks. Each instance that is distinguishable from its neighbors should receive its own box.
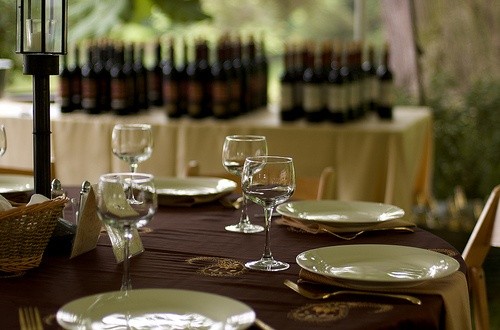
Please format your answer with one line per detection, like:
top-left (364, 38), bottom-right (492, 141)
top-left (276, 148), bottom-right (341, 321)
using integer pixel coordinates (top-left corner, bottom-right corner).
top-left (0, 185), bottom-right (475, 330)
top-left (0, 98), bottom-right (432, 229)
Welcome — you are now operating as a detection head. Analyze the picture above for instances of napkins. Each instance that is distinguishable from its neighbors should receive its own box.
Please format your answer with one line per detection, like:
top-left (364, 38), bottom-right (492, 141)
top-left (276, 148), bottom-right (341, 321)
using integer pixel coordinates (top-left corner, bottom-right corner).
top-left (275, 215), bottom-right (417, 235)
top-left (299, 268), bottom-right (472, 330)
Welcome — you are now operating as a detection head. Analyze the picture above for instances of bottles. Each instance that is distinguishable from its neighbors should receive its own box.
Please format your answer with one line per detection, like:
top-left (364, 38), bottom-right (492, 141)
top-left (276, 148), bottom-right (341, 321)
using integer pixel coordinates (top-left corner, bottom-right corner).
top-left (58, 29), bottom-right (268, 123)
top-left (78, 181), bottom-right (92, 222)
top-left (279, 38), bottom-right (394, 123)
top-left (50, 179), bottom-right (66, 219)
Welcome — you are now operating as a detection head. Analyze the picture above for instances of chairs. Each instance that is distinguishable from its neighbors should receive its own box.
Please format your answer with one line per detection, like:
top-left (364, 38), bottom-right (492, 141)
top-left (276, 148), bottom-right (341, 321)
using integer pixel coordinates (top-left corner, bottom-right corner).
top-left (462, 185), bottom-right (500, 330)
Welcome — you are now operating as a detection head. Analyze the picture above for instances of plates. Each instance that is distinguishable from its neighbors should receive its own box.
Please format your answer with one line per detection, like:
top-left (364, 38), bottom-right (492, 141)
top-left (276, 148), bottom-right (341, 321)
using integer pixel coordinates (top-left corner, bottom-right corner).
top-left (276, 199), bottom-right (405, 231)
top-left (0, 172), bottom-right (35, 195)
top-left (56, 288), bottom-right (256, 330)
top-left (150, 175), bottom-right (236, 208)
top-left (295, 244), bottom-right (460, 288)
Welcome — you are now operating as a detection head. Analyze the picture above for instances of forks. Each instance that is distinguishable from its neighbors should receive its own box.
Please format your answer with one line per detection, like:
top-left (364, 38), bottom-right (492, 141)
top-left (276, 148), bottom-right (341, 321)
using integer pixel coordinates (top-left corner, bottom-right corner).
top-left (283, 279), bottom-right (421, 306)
top-left (321, 226), bottom-right (414, 240)
top-left (18, 306), bottom-right (43, 330)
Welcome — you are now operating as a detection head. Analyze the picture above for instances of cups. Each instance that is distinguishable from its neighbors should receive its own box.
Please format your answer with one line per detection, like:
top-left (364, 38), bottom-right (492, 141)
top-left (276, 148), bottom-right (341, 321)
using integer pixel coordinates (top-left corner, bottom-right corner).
top-left (0, 124), bottom-right (7, 157)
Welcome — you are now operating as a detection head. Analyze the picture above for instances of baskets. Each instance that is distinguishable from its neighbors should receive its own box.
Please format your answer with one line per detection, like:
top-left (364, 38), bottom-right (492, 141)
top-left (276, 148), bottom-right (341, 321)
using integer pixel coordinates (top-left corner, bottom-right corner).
top-left (0, 192), bottom-right (71, 274)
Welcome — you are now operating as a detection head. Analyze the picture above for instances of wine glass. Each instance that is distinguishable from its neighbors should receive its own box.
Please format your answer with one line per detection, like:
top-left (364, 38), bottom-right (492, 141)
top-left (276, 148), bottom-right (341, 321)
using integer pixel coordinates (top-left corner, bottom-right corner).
top-left (240, 156), bottom-right (296, 272)
top-left (221, 135), bottom-right (268, 233)
top-left (110, 123), bottom-right (154, 204)
top-left (96, 173), bottom-right (157, 291)
